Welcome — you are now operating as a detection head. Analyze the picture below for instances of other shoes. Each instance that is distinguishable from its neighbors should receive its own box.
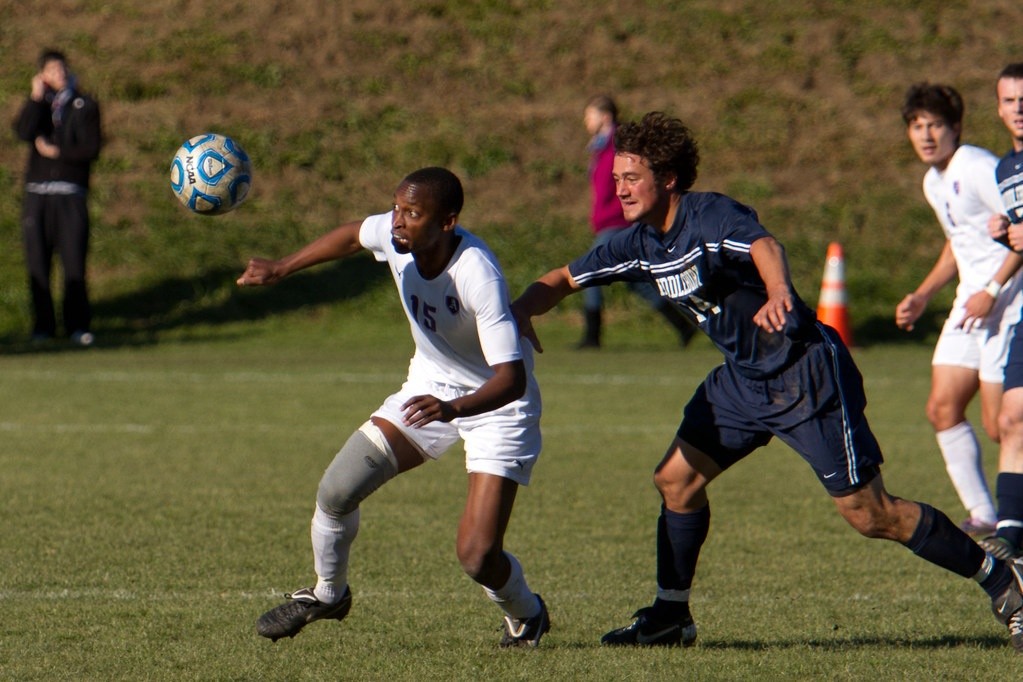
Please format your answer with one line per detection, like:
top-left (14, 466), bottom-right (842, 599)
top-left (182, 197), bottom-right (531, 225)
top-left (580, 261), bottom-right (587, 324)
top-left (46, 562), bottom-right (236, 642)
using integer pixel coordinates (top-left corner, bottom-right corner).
top-left (960, 519), bottom-right (998, 536)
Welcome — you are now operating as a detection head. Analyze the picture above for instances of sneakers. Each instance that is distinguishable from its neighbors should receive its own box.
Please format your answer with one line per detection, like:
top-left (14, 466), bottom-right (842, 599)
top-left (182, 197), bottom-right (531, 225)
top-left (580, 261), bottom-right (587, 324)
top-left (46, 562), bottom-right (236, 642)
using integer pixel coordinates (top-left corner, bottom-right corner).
top-left (496, 594), bottom-right (551, 648)
top-left (976, 534), bottom-right (1023, 560)
top-left (991, 559), bottom-right (1023, 649)
top-left (601, 607), bottom-right (697, 646)
top-left (256, 584), bottom-right (352, 642)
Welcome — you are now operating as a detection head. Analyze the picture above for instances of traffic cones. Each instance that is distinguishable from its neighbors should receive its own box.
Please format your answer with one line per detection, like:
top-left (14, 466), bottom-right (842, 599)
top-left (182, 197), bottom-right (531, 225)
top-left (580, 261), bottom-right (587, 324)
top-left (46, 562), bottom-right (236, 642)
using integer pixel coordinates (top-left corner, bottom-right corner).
top-left (812, 242), bottom-right (851, 344)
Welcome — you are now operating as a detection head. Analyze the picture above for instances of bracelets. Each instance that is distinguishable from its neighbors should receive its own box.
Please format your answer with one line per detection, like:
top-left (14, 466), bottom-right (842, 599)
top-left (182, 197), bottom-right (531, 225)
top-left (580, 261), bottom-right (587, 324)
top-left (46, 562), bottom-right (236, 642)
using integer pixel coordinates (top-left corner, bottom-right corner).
top-left (984, 278), bottom-right (1002, 298)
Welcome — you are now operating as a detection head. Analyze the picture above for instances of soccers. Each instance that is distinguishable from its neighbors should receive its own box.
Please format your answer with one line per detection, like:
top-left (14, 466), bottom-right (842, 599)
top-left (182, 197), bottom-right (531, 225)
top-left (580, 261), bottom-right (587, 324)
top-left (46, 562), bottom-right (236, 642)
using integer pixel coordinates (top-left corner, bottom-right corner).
top-left (169, 134), bottom-right (255, 216)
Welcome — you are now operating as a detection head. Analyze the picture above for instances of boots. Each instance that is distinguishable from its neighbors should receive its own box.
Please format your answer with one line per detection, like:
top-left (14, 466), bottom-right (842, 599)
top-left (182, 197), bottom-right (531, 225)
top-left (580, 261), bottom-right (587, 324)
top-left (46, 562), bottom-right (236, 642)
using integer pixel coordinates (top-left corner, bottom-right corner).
top-left (653, 298), bottom-right (698, 345)
top-left (575, 303), bottom-right (604, 348)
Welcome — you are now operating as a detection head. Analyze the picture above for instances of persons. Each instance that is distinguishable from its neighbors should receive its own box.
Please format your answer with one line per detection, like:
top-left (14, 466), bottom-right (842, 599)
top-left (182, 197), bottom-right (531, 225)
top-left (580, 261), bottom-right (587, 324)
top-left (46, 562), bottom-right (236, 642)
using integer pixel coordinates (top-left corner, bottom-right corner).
top-left (575, 92), bottom-right (698, 351)
top-left (977, 62), bottom-right (1023, 571)
top-left (894, 80), bottom-right (1022, 537)
top-left (10, 48), bottom-right (103, 347)
top-left (234, 166), bottom-right (553, 651)
top-left (509, 109), bottom-right (1023, 651)
